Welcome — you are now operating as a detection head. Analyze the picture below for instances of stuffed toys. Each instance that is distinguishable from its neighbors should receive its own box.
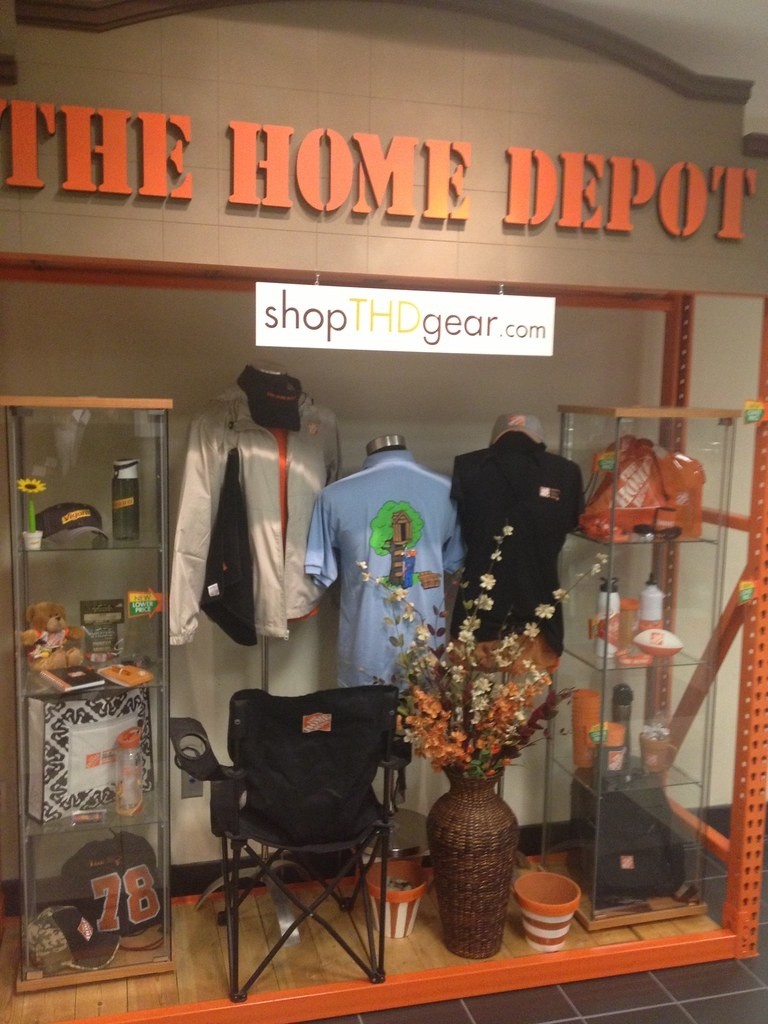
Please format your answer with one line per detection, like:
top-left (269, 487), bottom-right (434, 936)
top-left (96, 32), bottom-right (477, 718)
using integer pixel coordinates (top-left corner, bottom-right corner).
top-left (20, 602), bottom-right (83, 672)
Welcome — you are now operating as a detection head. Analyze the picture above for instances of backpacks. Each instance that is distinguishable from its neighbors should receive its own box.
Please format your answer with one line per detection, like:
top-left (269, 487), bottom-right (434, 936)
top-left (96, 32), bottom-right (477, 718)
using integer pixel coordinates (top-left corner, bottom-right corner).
top-left (569, 766), bottom-right (688, 909)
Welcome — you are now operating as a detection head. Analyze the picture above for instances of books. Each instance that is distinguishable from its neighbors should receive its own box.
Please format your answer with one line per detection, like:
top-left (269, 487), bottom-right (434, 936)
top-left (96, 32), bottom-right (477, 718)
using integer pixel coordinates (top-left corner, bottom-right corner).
top-left (40, 664), bottom-right (153, 692)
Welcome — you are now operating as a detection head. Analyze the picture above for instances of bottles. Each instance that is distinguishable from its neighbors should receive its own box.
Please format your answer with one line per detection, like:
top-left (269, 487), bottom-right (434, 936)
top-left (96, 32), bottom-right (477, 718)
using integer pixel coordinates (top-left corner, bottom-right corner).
top-left (638, 572), bottom-right (666, 632)
top-left (115, 726), bottom-right (143, 819)
top-left (596, 576), bottom-right (620, 659)
top-left (113, 458), bottom-right (142, 541)
top-left (618, 597), bottom-right (641, 650)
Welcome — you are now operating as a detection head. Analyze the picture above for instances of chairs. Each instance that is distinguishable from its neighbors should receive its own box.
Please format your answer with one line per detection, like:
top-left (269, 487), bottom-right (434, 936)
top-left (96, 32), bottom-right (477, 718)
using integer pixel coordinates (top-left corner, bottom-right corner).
top-left (209, 684), bottom-right (401, 1002)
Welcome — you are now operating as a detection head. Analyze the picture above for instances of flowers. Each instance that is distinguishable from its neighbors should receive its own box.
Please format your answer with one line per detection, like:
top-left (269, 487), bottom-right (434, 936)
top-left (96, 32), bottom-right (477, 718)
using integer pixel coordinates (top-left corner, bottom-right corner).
top-left (15, 478), bottom-right (48, 533)
top-left (354, 524), bottom-right (608, 782)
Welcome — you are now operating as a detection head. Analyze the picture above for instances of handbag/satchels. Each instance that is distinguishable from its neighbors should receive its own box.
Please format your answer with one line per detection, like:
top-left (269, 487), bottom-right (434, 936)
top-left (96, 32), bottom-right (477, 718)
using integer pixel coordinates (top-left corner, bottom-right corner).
top-left (578, 435), bottom-right (705, 539)
top-left (24, 679), bottom-right (159, 823)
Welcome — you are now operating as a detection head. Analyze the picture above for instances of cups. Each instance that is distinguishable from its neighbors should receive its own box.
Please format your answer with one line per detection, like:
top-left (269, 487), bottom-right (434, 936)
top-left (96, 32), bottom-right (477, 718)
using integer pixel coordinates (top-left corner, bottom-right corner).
top-left (595, 744), bottom-right (627, 780)
top-left (596, 721), bottom-right (624, 747)
top-left (572, 690), bottom-right (602, 768)
top-left (640, 731), bottom-right (678, 774)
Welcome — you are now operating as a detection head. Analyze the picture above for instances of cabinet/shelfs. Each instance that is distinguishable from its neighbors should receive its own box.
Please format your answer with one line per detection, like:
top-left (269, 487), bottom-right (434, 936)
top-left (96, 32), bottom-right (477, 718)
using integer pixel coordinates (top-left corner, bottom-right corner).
top-left (0, 396), bottom-right (178, 993)
top-left (552, 403), bottom-right (744, 936)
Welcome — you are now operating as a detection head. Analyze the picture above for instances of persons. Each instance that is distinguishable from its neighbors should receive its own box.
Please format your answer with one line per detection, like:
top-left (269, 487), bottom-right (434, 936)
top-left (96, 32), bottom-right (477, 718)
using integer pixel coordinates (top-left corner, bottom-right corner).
top-left (302, 434), bottom-right (469, 697)
top-left (176, 373), bottom-right (340, 644)
top-left (449, 412), bottom-right (586, 657)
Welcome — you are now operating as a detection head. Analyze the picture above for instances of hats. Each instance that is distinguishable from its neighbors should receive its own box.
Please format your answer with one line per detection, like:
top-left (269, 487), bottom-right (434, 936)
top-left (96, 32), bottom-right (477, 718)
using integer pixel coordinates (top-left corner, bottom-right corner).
top-left (238, 363), bottom-right (303, 431)
top-left (26, 906), bottom-right (121, 975)
top-left (36, 503), bottom-right (107, 543)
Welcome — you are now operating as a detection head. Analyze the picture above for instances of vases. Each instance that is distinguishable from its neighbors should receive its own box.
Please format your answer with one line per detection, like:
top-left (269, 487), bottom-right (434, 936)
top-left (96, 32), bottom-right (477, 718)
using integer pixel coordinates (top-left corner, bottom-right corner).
top-left (363, 859), bottom-right (431, 941)
top-left (514, 871), bottom-right (582, 953)
top-left (425, 765), bottom-right (520, 962)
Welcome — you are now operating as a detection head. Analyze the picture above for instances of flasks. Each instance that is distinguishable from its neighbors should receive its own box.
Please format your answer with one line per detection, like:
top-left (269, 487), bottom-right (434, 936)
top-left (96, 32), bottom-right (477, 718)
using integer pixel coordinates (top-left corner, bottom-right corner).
top-left (613, 684), bottom-right (633, 766)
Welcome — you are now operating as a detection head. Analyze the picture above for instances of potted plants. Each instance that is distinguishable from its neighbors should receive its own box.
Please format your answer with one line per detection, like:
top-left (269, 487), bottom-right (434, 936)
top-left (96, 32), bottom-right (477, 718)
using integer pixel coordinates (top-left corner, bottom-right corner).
top-left (23, 531), bottom-right (44, 551)
